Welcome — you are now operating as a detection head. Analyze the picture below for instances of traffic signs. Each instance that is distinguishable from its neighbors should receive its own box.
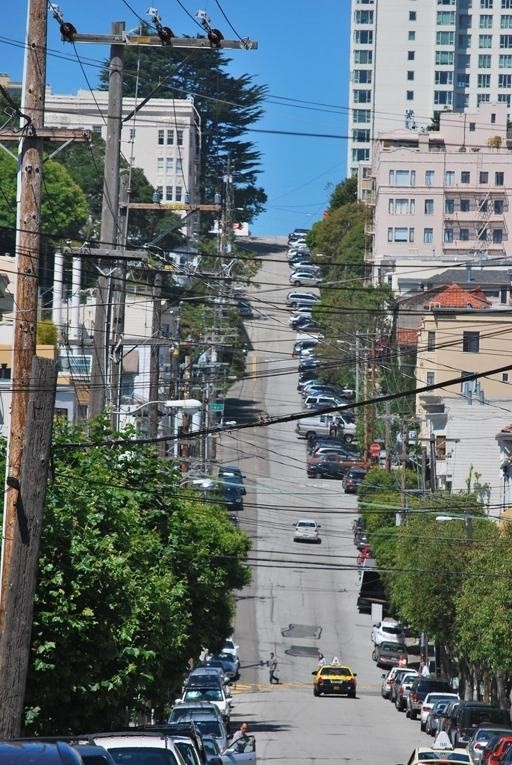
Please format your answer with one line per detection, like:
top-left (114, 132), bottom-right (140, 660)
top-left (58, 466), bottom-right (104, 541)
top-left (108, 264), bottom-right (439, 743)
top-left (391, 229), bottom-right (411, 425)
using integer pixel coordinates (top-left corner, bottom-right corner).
top-left (207, 402), bottom-right (224, 411)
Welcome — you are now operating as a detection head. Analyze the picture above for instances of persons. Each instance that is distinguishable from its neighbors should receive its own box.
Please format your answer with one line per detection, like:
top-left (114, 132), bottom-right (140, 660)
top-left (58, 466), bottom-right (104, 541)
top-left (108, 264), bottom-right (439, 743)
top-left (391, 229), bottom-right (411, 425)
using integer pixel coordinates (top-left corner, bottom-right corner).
top-left (330, 423), bottom-right (337, 437)
top-left (421, 662), bottom-right (431, 678)
top-left (318, 653), bottom-right (326, 665)
top-left (398, 655), bottom-right (407, 667)
top-left (233, 722), bottom-right (250, 753)
top-left (270, 652), bottom-right (279, 684)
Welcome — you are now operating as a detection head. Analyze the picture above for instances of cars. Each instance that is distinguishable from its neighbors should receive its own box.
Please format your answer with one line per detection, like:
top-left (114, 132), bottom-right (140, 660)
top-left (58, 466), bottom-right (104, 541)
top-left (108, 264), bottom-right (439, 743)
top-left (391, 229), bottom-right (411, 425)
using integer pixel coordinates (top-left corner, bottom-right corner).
top-left (311, 656), bottom-right (358, 698)
top-left (1, 632), bottom-right (258, 765)
top-left (352, 516), bottom-right (373, 563)
top-left (215, 464), bottom-right (248, 511)
top-left (292, 519), bottom-right (321, 541)
top-left (236, 297), bottom-right (254, 318)
top-left (287, 226), bottom-right (369, 492)
top-left (372, 615), bottom-right (512, 765)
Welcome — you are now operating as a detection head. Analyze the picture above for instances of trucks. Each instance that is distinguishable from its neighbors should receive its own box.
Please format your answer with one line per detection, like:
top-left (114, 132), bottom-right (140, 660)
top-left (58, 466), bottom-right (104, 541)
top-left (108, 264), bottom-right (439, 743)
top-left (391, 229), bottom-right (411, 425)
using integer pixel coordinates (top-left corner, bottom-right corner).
top-left (357, 557), bottom-right (397, 613)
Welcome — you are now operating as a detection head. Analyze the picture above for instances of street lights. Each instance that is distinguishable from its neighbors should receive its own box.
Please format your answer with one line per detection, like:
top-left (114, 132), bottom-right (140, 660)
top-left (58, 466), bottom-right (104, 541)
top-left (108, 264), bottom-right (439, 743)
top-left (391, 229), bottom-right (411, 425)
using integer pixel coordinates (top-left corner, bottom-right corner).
top-left (104, 396), bottom-right (204, 439)
top-left (204, 375), bottom-right (238, 464)
top-left (435, 515), bottom-right (473, 542)
